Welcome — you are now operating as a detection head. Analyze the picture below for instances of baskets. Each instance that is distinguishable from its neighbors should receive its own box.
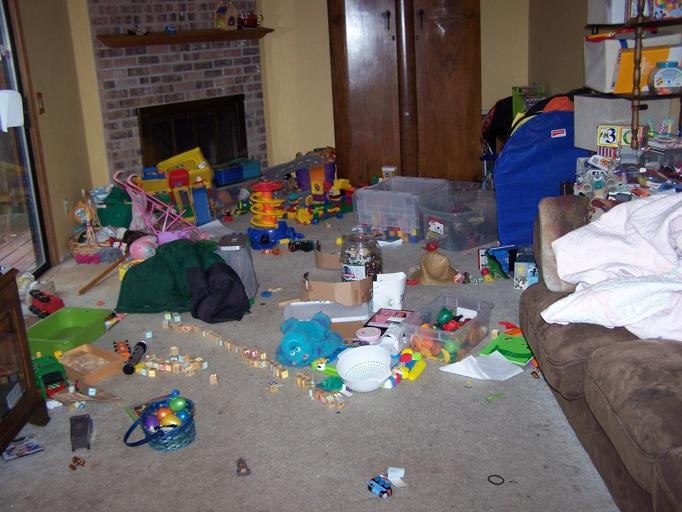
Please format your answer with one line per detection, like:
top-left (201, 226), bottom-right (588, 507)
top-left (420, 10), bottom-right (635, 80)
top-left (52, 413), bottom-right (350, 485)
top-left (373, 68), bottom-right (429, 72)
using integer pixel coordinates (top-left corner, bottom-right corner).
top-left (336, 344), bottom-right (392, 392)
top-left (138, 394), bottom-right (197, 452)
top-left (65, 226), bottom-right (113, 262)
top-left (134, 145), bottom-right (262, 196)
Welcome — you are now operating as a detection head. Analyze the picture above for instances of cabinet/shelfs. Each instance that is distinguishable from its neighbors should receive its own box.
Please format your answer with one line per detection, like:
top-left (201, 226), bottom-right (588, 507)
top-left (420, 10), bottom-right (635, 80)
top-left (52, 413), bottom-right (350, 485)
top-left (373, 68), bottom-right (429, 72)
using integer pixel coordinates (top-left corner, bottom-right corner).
top-left (1, 265), bottom-right (51, 457)
top-left (582, 1), bottom-right (682, 150)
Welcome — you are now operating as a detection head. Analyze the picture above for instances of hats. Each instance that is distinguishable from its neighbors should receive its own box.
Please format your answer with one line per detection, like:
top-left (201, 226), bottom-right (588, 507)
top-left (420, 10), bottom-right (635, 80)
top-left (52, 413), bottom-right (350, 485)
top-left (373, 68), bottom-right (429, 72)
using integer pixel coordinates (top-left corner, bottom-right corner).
top-left (411, 250), bottom-right (458, 286)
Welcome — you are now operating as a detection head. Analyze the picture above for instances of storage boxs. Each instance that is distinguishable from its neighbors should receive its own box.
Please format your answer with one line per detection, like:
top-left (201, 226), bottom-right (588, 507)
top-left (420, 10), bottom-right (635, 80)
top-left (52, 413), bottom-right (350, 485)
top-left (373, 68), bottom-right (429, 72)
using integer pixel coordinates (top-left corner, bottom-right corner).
top-left (355, 176), bottom-right (498, 253)
top-left (573, 32), bottom-right (682, 152)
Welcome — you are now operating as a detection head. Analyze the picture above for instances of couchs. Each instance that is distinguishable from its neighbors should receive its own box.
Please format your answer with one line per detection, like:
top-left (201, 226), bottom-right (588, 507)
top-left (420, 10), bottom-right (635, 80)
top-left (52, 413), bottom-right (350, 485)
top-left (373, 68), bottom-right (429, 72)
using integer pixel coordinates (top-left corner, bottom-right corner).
top-left (518, 195), bottom-right (680, 512)
